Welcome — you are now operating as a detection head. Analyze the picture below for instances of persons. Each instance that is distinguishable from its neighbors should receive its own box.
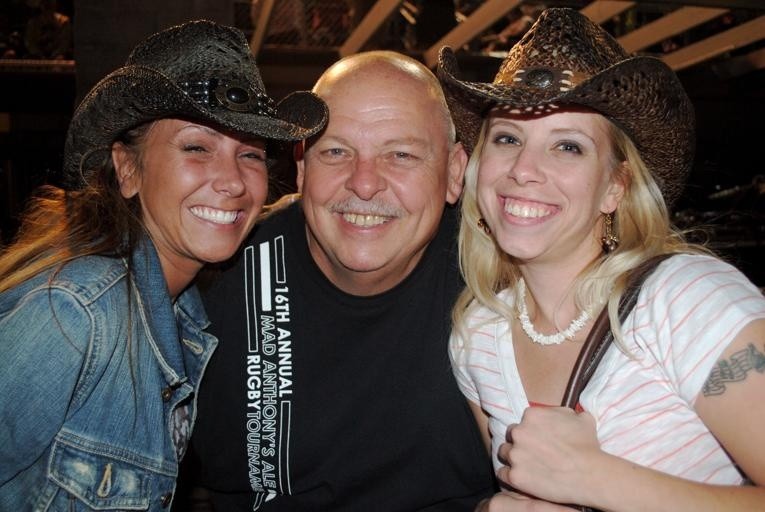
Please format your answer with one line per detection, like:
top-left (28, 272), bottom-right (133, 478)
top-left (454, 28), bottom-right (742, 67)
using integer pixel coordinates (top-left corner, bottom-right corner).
top-left (439, 7), bottom-right (765, 512)
top-left (172, 50), bottom-right (501, 512)
top-left (0, 19), bottom-right (330, 512)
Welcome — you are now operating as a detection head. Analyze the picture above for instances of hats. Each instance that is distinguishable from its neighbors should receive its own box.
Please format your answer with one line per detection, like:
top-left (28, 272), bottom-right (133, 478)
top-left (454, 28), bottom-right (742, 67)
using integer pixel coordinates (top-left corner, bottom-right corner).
top-left (435, 6), bottom-right (699, 215)
top-left (60, 19), bottom-right (330, 191)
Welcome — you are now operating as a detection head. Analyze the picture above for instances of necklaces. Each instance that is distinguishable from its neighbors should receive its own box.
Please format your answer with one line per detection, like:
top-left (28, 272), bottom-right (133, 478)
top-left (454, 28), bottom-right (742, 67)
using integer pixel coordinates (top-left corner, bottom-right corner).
top-left (515, 273), bottom-right (614, 344)
